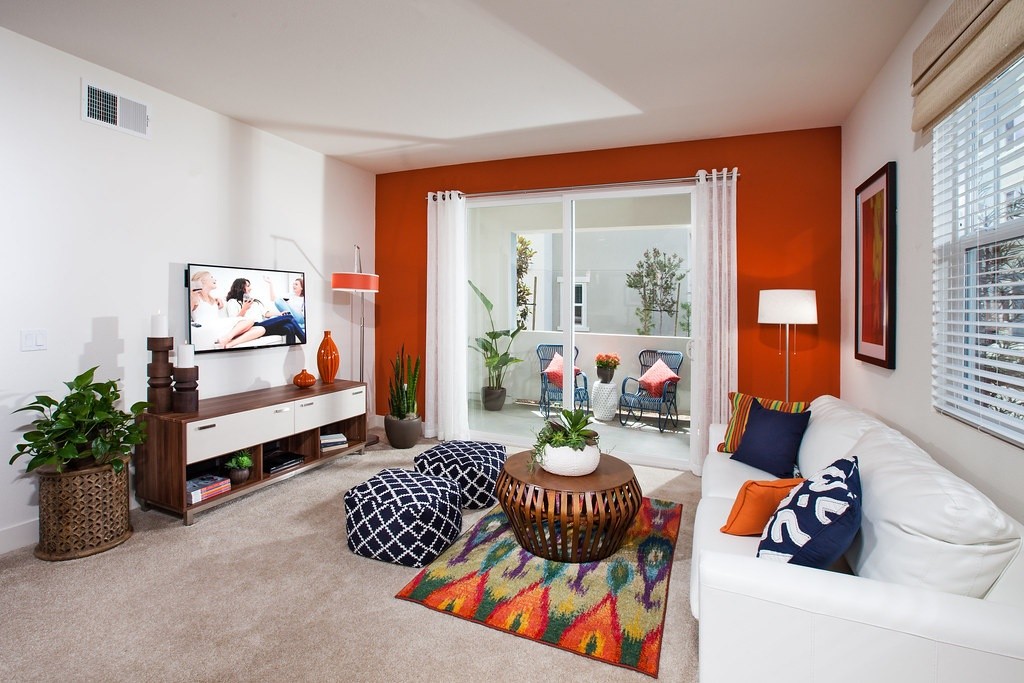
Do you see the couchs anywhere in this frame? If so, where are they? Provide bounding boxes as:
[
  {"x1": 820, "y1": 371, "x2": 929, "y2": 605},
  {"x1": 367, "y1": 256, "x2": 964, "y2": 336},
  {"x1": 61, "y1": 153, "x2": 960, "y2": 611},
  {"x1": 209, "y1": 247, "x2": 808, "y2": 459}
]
[{"x1": 689, "y1": 395, "x2": 1023, "y2": 683}]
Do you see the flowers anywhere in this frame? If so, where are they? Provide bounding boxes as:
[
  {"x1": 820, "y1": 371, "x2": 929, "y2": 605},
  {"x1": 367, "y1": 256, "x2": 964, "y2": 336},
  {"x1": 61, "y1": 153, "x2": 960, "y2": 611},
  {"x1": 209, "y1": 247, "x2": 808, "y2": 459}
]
[{"x1": 595, "y1": 353, "x2": 620, "y2": 367}]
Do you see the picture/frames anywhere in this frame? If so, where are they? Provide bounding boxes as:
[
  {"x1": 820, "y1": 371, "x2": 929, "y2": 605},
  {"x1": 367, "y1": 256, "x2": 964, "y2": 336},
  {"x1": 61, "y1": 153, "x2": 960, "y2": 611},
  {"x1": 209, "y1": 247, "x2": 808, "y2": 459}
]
[{"x1": 855, "y1": 161, "x2": 896, "y2": 370}]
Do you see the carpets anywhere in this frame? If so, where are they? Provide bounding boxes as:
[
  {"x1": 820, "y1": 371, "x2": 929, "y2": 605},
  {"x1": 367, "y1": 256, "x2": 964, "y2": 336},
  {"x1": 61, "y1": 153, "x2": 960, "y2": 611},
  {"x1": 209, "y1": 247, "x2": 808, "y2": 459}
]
[{"x1": 393, "y1": 495, "x2": 683, "y2": 679}]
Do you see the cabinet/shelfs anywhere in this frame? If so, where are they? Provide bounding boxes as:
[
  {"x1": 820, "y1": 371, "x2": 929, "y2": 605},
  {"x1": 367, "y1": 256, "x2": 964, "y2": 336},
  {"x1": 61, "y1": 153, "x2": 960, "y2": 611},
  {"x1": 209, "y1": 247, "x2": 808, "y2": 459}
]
[{"x1": 135, "y1": 378, "x2": 368, "y2": 526}]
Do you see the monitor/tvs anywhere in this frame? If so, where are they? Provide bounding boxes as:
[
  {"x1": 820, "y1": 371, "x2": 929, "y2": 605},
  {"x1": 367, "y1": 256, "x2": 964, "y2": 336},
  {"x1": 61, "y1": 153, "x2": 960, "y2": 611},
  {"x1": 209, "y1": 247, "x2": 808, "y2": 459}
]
[{"x1": 187, "y1": 262, "x2": 306, "y2": 354}]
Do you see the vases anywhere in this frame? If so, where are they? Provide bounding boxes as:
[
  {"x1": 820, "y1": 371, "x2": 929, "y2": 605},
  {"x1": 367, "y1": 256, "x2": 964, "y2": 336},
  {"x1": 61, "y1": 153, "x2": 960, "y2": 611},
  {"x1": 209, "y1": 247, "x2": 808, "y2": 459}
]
[{"x1": 597, "y1": 366, "x2": 614, "y2": 382}]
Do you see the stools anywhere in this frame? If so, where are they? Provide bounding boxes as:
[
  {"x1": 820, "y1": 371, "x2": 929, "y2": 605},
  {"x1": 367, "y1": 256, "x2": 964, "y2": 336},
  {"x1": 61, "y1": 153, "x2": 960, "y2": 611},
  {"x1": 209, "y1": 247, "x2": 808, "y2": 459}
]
[
  {"x1": 344, "y1": 467, "x2": 462, "y2": 568},
  {"x1": 413, "y1": 439, "x2": 507, "y2": 509}
]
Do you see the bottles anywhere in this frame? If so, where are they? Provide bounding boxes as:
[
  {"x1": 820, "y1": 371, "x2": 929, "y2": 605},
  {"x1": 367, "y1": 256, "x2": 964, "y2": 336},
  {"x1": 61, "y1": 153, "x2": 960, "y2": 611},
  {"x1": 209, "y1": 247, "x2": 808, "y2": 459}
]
[
  {"x1": 317, "y1": 331, "x2": 340, "y2": 385},
  {"x1": 293, "y1": 369, "x2": 316, "y2": 388}
]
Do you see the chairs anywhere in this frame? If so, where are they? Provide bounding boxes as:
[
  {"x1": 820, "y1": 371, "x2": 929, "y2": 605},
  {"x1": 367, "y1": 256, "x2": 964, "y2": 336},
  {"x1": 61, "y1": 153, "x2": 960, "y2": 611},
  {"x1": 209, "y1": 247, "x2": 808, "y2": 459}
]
[
  {"x1": 536, "y1": 343, "x2": 589, "y2": 420},
  {"x1": 619, "y1": 350, "x2": 684, "y2": 432}
]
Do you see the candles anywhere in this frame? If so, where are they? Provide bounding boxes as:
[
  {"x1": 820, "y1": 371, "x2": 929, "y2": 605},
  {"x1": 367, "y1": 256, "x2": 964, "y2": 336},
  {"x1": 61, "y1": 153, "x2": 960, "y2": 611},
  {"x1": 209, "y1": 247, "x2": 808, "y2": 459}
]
[
  {"x1": 151, "y1": 312, "x2": 168, "y2": 338},
  {"x1": 177, "y1": 339, "x2": 195, "y2": 368}
]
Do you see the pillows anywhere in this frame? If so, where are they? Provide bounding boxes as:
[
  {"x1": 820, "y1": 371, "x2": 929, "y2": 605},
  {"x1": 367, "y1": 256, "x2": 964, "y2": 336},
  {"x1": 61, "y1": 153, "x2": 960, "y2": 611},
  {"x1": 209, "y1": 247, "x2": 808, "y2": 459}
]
[
  {"x1": 542, "y1": 352, "x2": 580, "y2": 390},
  {"x1": 717, "y1": 391, "x2": 809, "y2": 453},
  {"x1": 720, "y1": 478, "x2": 805, "y2": 536},
  {"x1": 729, "y1": 397, "x2": 811, "y2": 479},
  {"x1": 637, "y1": 358, "x2": 681, "y2": 398},
  {"x1": 756, "y1": 456, "x2": 862, "y2": 570}
]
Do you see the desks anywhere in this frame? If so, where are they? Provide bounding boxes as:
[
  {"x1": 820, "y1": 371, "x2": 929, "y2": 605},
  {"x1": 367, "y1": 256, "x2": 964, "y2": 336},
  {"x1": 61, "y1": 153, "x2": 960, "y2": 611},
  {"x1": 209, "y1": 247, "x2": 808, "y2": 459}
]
[
  {"x1": 591, "y1": 380, "x2": 618, "y2": 421},
  {"x1": 31, "y1": 451, "x2": 134, "y2": 562},
  {"x1": 496, "y1": 450, "x2": 643, "y2": 563}
]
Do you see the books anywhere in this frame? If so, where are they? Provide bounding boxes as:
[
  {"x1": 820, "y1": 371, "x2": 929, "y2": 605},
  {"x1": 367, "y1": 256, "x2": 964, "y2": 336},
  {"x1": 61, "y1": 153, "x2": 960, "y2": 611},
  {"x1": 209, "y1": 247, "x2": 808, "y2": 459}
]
[
  {"x1": 186, "y1": 474, "x2": 232, "y2": 505},
  {"x1": 263, "y1": 447, "x2": 305, "y2": 474},
  {"x1": 320, "y1": 433, "x2": 348, "y2": 452}
]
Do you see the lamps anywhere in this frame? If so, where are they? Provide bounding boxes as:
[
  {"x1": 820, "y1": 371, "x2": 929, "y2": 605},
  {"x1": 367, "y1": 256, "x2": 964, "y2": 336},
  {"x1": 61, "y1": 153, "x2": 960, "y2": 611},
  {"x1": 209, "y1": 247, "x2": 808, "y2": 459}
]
[
  {"x1": 331, "y1": 243, "x2": 380, "y2": 447},
  {"x1": 757, "y1": 288, "x2": 818, "y2": 402}
]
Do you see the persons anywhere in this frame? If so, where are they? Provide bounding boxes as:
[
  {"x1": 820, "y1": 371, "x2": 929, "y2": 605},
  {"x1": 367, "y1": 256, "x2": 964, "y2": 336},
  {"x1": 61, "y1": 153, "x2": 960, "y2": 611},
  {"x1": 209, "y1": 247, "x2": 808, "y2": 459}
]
[
  {"x1": 226, "y1": 278, "x2": 306, "y2": 345},
  {"x1": 263, "y1": 275, "x2": 305, "y2": 329},
  {"x1": 190, "y1": 271, "x2": 266, "y2": 349}
]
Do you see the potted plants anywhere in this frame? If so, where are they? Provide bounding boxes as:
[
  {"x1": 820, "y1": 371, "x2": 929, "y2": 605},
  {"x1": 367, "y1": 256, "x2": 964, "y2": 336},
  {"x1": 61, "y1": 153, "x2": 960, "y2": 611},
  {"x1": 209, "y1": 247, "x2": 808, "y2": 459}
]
[
  {"x1": 467, "y1": 280, "x2": 525, "y2": 412},
  {"x1": 224, "y1": 448, "x2": 254, "y2": 483},
  {"x1": 383, "y1": 343, "x2": 422, "y2": 449},
  {"x1": 8, "y1": 364, "x2": 160, "y2": 474},
  {"x1": 525, "y1": 408, "x2": 601, "y2": 478}
]
